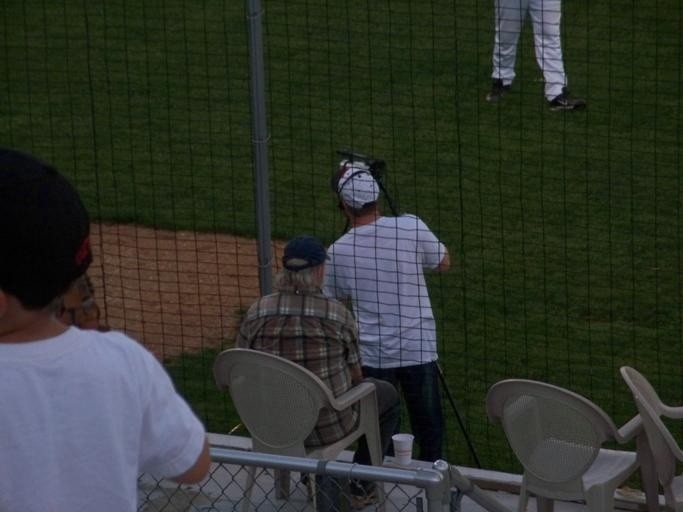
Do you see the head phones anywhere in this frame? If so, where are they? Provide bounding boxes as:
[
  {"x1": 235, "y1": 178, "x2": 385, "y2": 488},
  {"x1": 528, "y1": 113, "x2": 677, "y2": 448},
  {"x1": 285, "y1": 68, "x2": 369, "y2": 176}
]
[{"x1": 336, "y1": 171, "x2": 368, "y2": 210}]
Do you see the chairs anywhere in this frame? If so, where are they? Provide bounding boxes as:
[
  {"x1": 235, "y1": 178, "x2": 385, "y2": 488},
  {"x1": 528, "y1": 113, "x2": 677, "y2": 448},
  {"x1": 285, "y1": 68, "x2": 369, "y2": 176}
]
[
  {"x1": 484, "y1": 366, "x2": 683, "y2": 511},
  {"x1": 212, "y1": 344, "x2": 387, "y2": 512}
]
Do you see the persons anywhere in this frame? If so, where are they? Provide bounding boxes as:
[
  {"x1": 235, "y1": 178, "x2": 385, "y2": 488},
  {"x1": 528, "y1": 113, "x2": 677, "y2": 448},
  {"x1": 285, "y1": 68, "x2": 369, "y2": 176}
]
[
  {"x1": 235, "y1": 234, "x2": 402, "y2": 510},
  {"x1": 0, "y1": 148, "x2": 213, "y2": 512},
  {"x1": 484, "y1": 1, "x2": 588, "y2": 116},
  {"x1": 319, "y1": 155, "x2": 453, "y2": 465}
]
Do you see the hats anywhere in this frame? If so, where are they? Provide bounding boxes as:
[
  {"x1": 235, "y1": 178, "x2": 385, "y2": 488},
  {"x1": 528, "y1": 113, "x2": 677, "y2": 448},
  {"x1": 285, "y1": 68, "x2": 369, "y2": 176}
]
[
  {"x1": 339, "y1": 167, "x2": 380, "y2": 209},
  {"x1": 281, "y1": 234, "x2": 332, "y2": 270},
  {"x1": 0, "y1": 151, "x2": 93, "y2": 291}
]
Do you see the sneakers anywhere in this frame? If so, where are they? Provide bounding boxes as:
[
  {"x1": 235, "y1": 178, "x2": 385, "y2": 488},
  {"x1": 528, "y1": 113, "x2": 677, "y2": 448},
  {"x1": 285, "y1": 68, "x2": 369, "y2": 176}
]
[
  {"x1": 347, "y1": 480, "x2": 379, "y2": 512},
  {"x1": 487, "y1": 78, "x2": 511, "y2": 101},
  {"x1": 548, "y1": 88, "x2": 586, "y2": 111}
]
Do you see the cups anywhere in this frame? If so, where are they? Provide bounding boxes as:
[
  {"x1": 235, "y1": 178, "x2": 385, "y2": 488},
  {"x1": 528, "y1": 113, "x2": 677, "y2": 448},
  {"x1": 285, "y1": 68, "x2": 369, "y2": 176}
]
[{"x1": 391, "y1": 433, "x2": 415, "y2": 466}]
